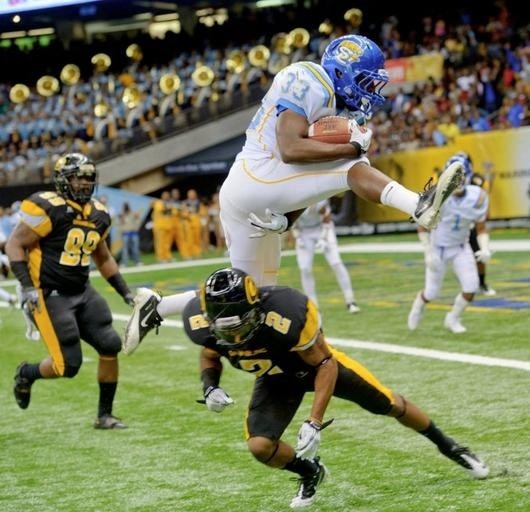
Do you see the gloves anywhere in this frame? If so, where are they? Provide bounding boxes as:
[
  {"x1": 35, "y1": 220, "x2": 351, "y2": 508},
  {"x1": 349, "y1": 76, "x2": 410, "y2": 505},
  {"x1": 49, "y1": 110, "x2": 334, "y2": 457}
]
[
  {"x1": 417, "y1": 232, "x2": 442, "y2": 271},
  {"x1": 350, "y1": 120, "x2": 373, "y2": 154},
  {"x1": 11, "y1": 260, "x2": 40, "y2": 311},
  {"x1": 248, "y1": 208, "x2": 288, "y2": 239},
  {"x1": 196, "y1": 386, "x2": 234, "y2": 412},
  {"x1": 316, "y1": 222, "x2": 334, "y2": 254},
  {"x1": 107, "y1": 274, "x2": 135, "y2": 306},
  {"x1": 474, "y1": 233, "x2": 492, "y2": 263},
  {"x1": 291, "y1": 226, "x2": 306, "y2": 250},
  {"x1": 296, "y1": 417, "x2": 334, "y2": 462}
]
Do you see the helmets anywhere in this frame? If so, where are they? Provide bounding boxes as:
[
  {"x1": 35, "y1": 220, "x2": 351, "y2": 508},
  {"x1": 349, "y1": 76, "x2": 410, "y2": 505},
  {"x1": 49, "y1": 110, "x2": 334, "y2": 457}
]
[
  {"x1": 321, "y1": 35, "x2": 389, "y2": 117},
  {"x1": 201, "y1": 267, "x2": 262, "y2": 346},
  {"x1": 445, "y1": 156, "x2": 472, "y2": 182},
  {"x1": 53, "y1": 152, "x2": 96, "y2": 204}
]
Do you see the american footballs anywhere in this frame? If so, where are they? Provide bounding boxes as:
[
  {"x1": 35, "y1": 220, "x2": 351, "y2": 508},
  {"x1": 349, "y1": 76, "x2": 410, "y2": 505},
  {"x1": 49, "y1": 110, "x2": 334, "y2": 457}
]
[{"x1": 307, "y1": 116, "x2": 364, "y2": 144}]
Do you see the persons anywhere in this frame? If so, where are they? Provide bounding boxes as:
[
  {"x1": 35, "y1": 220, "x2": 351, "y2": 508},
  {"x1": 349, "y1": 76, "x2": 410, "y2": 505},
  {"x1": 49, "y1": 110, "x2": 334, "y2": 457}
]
[
  {"x1": 0, "y1": 0, "x2": 530, "y2": 307},
  {"x1": 408, "y1": 150, "x2": 492, "y2": 334},
  {"x1": 180, "y1": 267, "x2": 489, "y2": 508},
  {"x1": 5, "y1": 151, "x2": 134, "y2": 429},
  {"x1": 291, "y1": 198, "x2": 361, "y2": 319},
  {"x1": 120, "y1": 31, "x2": 471, "y2": 359}
]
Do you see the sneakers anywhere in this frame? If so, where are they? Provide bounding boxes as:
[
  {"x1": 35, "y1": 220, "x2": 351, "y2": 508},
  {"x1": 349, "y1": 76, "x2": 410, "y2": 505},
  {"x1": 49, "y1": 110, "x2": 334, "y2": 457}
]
[
  {"x1": 408, "y1": 291, "x2": 425, "y2": 330},
  {"x1": 448, "y1": 444, "x2": 491, "y2": 480},
  {"x1": 443, "y1": 314, "x2": 465, "y2": 333},
  {"x1": 94, "y1": 415, "x2": 128, "y2": 429},
  {"x1": 347, "y1": 304, "x2": 360, "y2": 312},
  {"x1": 289, "y1": 456, "x2": 329, "y2": 507},
  {"x1": 412, "y1": 162, "x2": 466, "y2": 229},
  {"x1": 124, "y1": 287, "x2": 163, "y2": 356},
  {"x1": 14, "y1": 361, "x2": 33, "y2": 409},
  {"x1": 482, "y1": 289, "x2": 495, "y2": 297}
]
